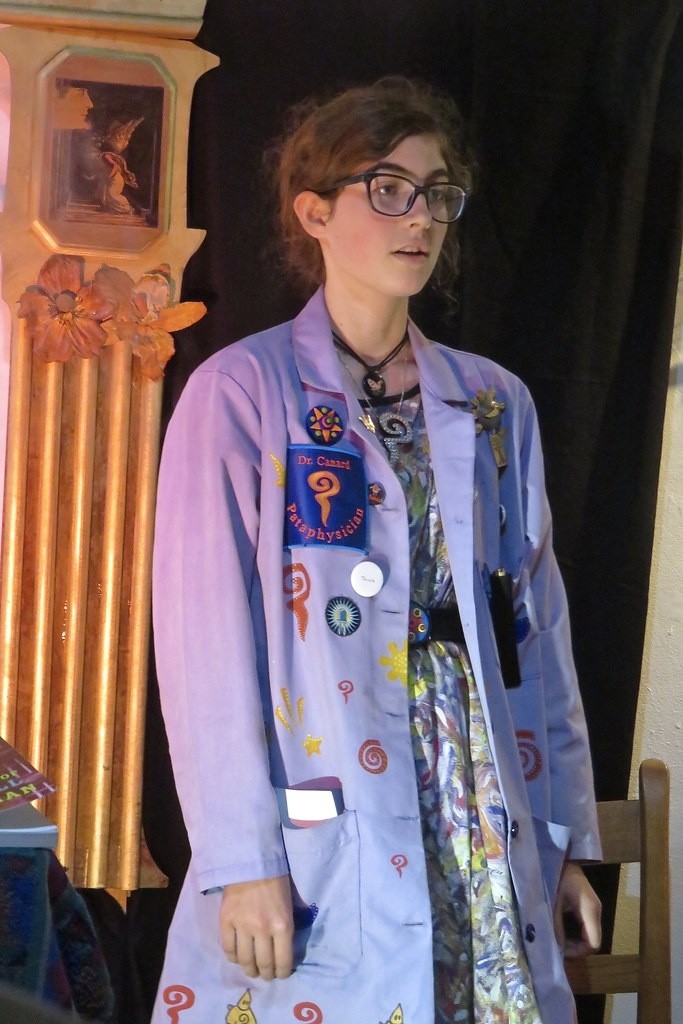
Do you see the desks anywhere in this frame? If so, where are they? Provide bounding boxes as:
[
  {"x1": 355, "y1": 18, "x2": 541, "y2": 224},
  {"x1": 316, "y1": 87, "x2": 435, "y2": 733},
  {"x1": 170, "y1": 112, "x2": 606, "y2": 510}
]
[{"x1": 0, "y1": 803, "x2": 59, "y2": 1024}]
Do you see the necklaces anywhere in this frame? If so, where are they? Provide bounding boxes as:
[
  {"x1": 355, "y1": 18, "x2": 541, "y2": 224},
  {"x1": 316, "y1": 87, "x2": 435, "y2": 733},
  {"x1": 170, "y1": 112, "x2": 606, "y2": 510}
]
[{"x1": 331, "y1": 330, "x2": 413, "y2": 470}]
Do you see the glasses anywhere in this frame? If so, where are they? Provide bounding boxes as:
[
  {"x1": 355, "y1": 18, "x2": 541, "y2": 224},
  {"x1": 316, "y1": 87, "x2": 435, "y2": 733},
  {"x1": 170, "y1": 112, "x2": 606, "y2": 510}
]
[{"x1": 325, "y1": 171, "x2": 466, "y2": 224}]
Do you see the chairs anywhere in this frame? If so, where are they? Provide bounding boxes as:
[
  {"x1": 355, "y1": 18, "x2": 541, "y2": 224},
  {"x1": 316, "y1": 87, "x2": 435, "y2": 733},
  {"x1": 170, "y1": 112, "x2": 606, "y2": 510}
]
[{"x1": 560, "y1": 757, "x2": 674, "y2": 1024}]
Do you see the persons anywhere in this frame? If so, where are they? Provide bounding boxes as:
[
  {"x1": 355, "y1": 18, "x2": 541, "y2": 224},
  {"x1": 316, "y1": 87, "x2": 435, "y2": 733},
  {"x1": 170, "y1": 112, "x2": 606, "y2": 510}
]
[{"x1": 149, "y1": 74, "x2": 603, "y2": 1024}]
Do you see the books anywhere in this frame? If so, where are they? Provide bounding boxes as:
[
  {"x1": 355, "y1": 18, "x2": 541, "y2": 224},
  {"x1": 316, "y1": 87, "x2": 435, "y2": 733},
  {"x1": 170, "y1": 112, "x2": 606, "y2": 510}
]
[{"x1": 0, "y1": 730, "x2": 60, "y2": 849}]
